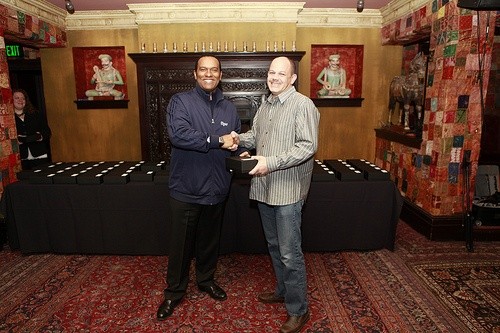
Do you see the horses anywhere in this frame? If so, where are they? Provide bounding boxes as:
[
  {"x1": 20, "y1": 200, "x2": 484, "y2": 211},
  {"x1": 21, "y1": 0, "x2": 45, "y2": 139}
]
[{"x1": 388, "y1": 51, "x2": 426, "y2": 130}]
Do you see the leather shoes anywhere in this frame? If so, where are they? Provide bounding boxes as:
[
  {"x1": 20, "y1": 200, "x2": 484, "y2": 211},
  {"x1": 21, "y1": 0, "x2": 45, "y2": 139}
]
[
  {"x1": 278, "y1": 311, "x2": 309, "y2": 333},
  {"x1": 156, "y1": 298, "x2": 182, "y2": 320},
  {"x1": 258, "y1": 293, "x2": 285, "y2": 303},
  {"x1": 197, "y1": 283, "x2": 227, "y2": 301}
]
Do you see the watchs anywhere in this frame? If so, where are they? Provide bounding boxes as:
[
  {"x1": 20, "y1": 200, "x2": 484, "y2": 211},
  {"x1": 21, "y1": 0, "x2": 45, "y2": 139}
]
[{"x1": 218, "y1": 136, "x2": 224, "y2": 147}]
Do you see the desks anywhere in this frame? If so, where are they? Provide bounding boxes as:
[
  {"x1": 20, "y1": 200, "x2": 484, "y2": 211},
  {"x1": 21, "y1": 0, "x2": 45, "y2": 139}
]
[{"x1": 4, "y1": 159, "x2": 404, "y2": 255}]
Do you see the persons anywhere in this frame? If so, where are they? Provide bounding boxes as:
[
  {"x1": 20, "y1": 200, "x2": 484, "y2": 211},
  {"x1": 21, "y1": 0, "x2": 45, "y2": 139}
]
[
  {"x1": 157, "y1": 55, "x2": 253, "y2": 320},
  {"x1": 13, "y1": 90, "x2": 52, "y2": 170},
  {"x1": 85, "y1": 54, "x2": 125, "y2": 100},
  {"x1": 316, "y1": 54, "x2": 352, "y2": 98},
  {"x1": 227, "y1": 56, "x2": 321, "y2": 333}
]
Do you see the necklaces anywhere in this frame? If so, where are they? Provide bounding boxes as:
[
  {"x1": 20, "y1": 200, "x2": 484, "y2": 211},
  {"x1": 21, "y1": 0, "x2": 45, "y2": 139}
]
[{"x1": 16, "y1": 112, "x2": 24, "y2": 117}]
